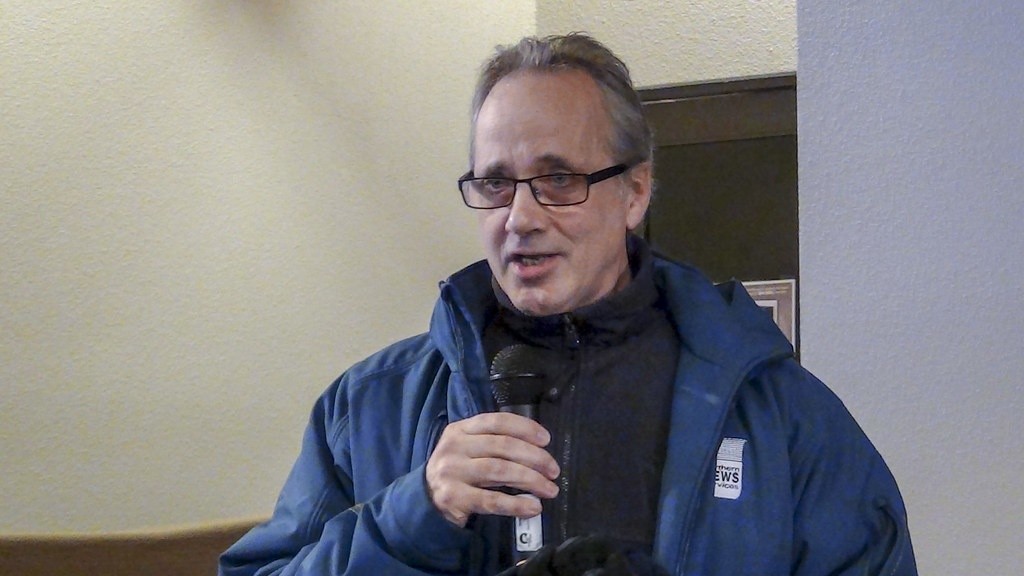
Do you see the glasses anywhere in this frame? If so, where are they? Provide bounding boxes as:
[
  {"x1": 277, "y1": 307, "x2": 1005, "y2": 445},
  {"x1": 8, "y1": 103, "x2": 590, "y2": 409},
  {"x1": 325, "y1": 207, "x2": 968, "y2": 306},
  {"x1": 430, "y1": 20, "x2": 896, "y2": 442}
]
[{"x1": 458, "y1": 159, "x2": 639, "y2": 209}]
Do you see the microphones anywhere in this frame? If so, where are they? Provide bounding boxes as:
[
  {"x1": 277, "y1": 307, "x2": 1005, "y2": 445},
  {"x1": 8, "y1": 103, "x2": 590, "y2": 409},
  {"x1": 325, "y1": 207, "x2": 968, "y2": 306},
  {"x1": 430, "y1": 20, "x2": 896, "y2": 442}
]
[{"x1": 492, "y1": 345, "x2": 548, "y2": 560}]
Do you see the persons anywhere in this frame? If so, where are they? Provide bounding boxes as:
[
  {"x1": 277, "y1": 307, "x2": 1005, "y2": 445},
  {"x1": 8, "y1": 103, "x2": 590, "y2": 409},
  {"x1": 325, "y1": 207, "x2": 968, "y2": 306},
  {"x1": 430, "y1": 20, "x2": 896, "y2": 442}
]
[{"x1": 217, "y1": 33, "x2": 918, "y2": 576}]
